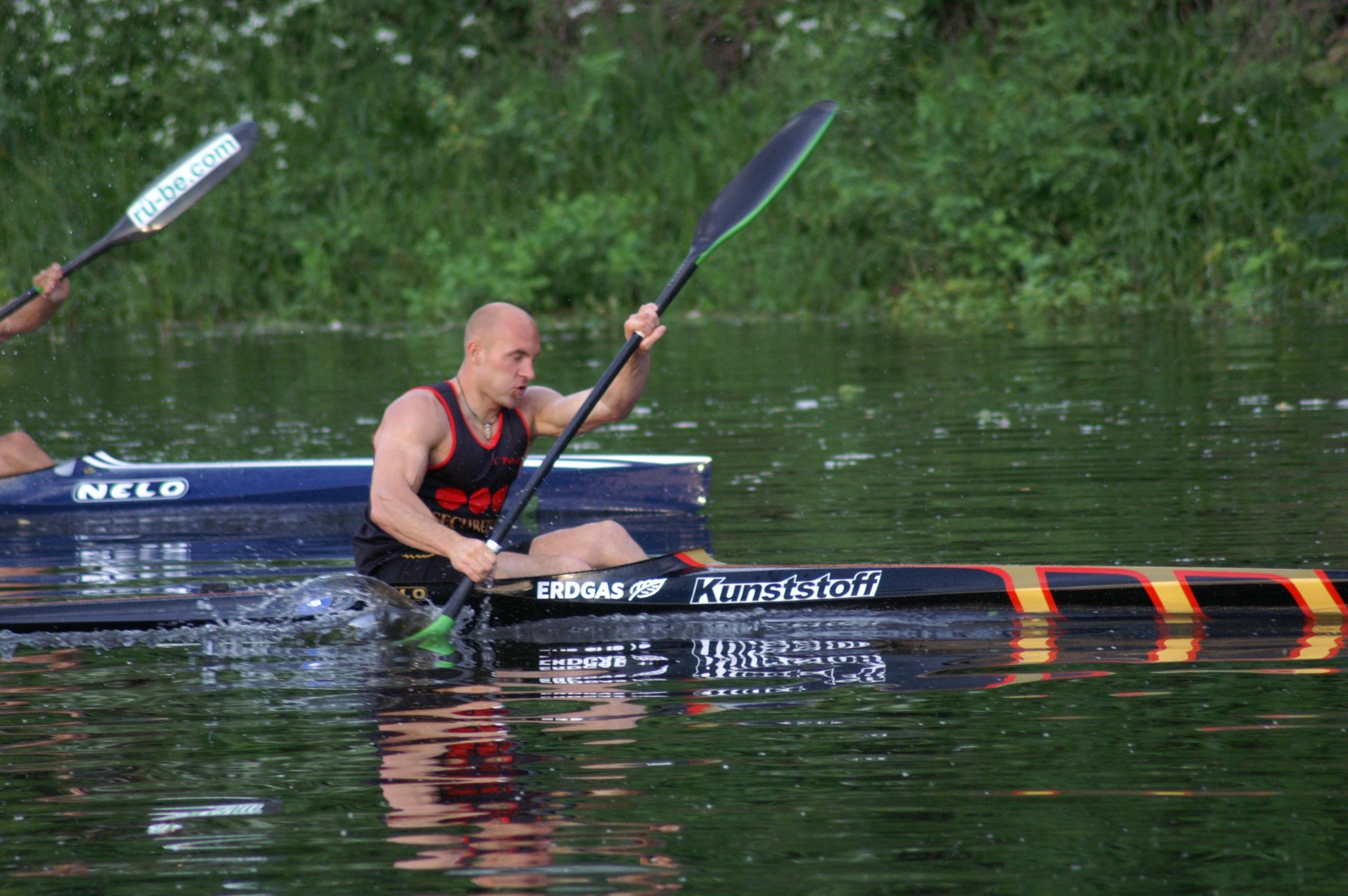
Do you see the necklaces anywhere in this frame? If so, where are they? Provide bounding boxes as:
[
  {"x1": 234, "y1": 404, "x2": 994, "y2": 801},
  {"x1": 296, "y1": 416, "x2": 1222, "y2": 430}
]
[{"x1": 457, "y1": 375, "x2": 502, "y2": 440}]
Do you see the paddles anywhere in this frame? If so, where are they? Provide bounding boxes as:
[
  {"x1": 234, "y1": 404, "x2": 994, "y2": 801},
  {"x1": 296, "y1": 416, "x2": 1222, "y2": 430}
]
[
  {"x1": 0, "y1": 116, "x2": 264, "y2": 319},
  {"x1": 391, "y1": 94, "x2": 843, "y2": 640}
]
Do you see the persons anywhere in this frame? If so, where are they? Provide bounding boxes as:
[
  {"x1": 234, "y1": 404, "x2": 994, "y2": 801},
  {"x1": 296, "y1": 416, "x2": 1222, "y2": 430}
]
[
  {"x1": 0, "y1": 262, "x2": 71, "y2": 477},
  {"x1": 351, "y1": 299, "x2": 667, "y2": 616}
]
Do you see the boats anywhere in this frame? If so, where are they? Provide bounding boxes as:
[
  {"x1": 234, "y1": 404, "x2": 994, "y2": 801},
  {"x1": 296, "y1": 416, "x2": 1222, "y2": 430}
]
[
  {"x1": 0, "y1": 544, "x2": 1348, "y2": 619},
  {"x1": 0, "y1": 446, "x2": 713, "y2": 510}
]
